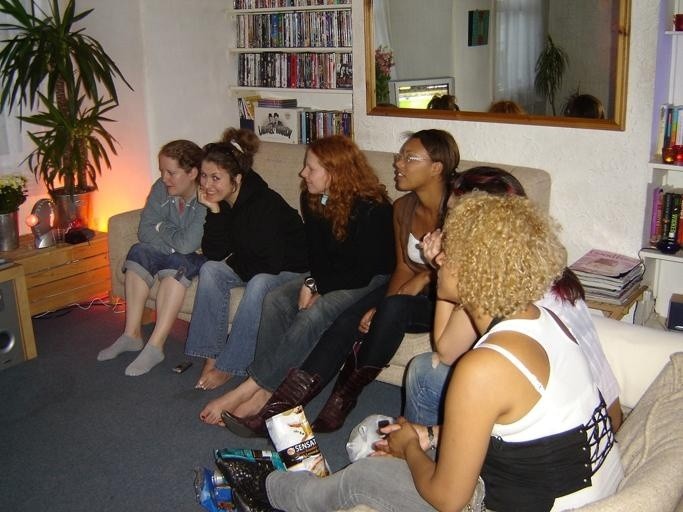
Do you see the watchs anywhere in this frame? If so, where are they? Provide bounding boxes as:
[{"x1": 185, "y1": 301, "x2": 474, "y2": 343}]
[{"x1": 305, "y1": 275, "x2": 318, "y2": 294}]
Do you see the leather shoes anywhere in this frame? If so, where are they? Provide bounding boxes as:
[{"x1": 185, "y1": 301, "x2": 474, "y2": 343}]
[{"x1": 215, "y1": 455, "x2": 286, "y2": 512}]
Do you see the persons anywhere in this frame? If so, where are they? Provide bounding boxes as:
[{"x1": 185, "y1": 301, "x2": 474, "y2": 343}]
[
  {"x1": 95, "y1": 138, "x2": 207, "y2": 378},
  {"x1": 180, "y1": 126, "x2": 317, "y2": 391},
  {"x1": 211, "y1": 191, "x2": 626, "y2": 511},
  {"x1": 427, "y1": 93, "x2": 461, "y2": 110},
  {"x1": 220, "y1": 128, "x2": 460, "y2": 441},
  {"x1": 378, "y1": 101, "x2": 398, "y2": 108},
  {"x1": 196, "y1": 132, "x2": 395, "y2": 429},
  {"x1": 485, "y1": 99, "x2": 529, "y2": 116},
  {"x1": 401, "y1": 166, "x2": 626, "y2": 437},
  {"x1": 561, "y1": 88, "x2": 607, "y2": 118}
]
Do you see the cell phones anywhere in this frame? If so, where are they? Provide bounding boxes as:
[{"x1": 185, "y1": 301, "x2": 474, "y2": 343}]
[{"x1": 172, "y1": 360, "x2": 193, "y2": 373}]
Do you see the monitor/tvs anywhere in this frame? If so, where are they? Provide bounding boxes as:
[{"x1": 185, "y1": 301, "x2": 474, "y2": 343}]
[{"x1": 389, "y1": 76, "x2": 455, "y2": 110}]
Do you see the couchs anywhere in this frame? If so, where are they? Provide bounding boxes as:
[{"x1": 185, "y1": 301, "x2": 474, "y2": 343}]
[{"x1": 105, "y1": 132, "x2": 551, "y2": 389}]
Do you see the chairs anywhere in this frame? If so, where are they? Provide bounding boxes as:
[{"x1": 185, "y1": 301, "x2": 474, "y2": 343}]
[{"x1": 575, "y1": 348, "x2": 681, "y2": 512}]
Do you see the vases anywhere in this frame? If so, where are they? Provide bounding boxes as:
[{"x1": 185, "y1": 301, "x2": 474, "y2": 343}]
[
  {"x1": 0, "y1": 212, "x2": 21, "y2": 252},
  {"x1": 375, "y1": 78, "x2": 389, "y2": 106}
]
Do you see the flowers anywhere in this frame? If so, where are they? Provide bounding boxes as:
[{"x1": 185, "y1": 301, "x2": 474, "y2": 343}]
[
  {"x1": 0, "y1": 174, "x2": 30, "y2": 214},
  {"x1": 373, "y1": 44, "x2": 394, "y2": 100}
]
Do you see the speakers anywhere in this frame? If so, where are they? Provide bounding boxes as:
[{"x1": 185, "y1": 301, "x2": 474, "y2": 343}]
[{"x1": 0, "y1": 257, "x2": 39, "y2": 373}]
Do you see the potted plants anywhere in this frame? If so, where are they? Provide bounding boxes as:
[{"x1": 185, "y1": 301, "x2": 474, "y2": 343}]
[{"x1": 0, "y1": 0, "x2": 134, "y2": 231}]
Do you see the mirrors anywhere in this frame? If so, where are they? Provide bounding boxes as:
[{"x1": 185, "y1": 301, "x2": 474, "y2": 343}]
[{"x1": 360, "y1": 1, "x2": 632, "y2": 134}]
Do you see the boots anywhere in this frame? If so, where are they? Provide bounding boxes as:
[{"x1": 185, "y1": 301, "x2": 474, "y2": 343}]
[
  {"x1": 221, "y1": 367, "x2": 327, "y2": 438},
  {"x1": 308, "y1": 343, "x2": 389, "y2": 434}
]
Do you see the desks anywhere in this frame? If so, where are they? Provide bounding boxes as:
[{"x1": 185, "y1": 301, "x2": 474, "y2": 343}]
[{"x1": 583, "y1": 283, "x2": 650, "y2": 323}]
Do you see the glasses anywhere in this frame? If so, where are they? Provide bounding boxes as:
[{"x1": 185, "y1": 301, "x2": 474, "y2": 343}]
[{"x1": 392, "y1": 153, "x2": 431, "y2": 164}]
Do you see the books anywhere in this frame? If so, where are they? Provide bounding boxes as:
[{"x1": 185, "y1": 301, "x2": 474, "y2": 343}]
[
  {"x1": 565, "y1": 100, "x2": 683, "y2": 330},
  {"x1": 232, "y1": 0, "x2": 354, "y2": 145}
]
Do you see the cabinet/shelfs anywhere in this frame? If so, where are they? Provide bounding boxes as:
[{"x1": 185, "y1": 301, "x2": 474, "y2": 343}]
[
  {"x1": 0, "y1": 228, "x2": 111, "y2": 319},
  {"x1": 230, "y1": 0, "x2": 354, "y2": 144},
  {"x1": 639, "y1": 156, "x2": 682, "y2": 330}
]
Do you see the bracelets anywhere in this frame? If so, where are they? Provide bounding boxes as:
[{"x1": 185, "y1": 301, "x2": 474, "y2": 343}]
[{"x1": 425, "y1": 423, "x2": 437, "y2": 450}]
